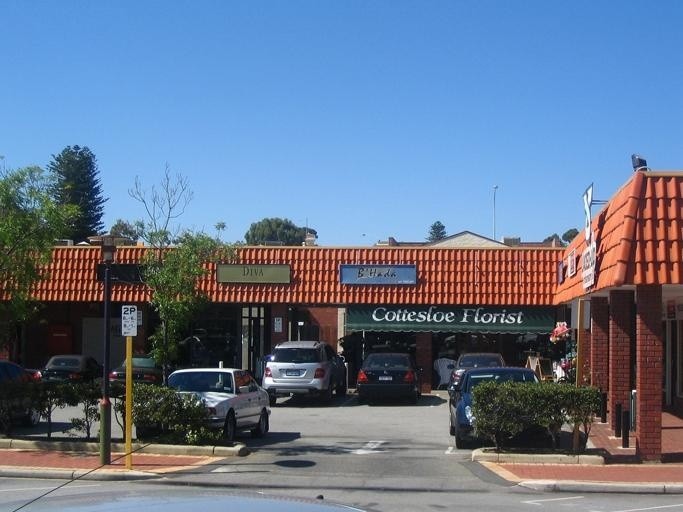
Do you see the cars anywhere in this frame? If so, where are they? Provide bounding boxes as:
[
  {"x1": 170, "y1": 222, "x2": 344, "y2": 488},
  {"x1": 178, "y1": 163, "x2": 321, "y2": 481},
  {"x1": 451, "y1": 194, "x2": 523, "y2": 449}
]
[
  {"x1": 260, "y1": 338, "x2": 426, "y2": 408},
  {"x1": 448, "y1": 350, "x2": 548, "y2": 452},
  {"x1": 147, "y1": 366, "x2": 270, "y2": 445},
  {"x1": 44, "y1": 354, "x2": 164, "y2": 390},
  {"x1": 0, "y1": 362, "x2": 45, "y2": 426}
]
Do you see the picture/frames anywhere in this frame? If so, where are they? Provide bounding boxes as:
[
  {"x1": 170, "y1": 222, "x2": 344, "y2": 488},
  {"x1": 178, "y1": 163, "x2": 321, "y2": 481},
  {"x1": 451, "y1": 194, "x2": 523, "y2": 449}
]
[{"x1": 567, "y1": 248, "x2": 577, "y2": 278}]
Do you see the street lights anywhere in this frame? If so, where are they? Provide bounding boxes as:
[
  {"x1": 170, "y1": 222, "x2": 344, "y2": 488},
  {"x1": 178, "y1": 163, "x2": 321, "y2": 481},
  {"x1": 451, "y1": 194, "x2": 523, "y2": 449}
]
[
  {"x1": 492, "y1": 185, "x2": 499, "y2": 240},
  {"x1": 100, "y1": 234, "x2": 116, "y2": 464}
]
